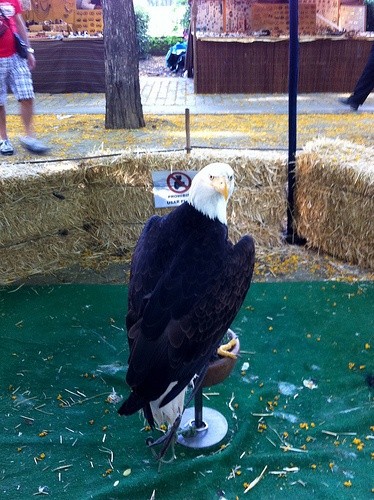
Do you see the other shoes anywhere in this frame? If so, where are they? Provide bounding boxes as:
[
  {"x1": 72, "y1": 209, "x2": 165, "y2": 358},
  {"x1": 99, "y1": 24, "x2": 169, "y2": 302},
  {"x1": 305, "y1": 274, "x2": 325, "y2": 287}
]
[{"x1": 338, "y1": 97, "x2": 359, "y2": 111}]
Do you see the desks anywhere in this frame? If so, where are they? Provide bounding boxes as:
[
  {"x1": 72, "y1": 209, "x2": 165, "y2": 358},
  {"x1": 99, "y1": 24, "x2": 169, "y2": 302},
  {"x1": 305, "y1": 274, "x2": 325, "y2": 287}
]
[
  {"x1": 195, "y1": 39, "x2": 374, "y2": 92},
  {"x1": 30, "y1": 40, "x2": 104, "y2": 94}
]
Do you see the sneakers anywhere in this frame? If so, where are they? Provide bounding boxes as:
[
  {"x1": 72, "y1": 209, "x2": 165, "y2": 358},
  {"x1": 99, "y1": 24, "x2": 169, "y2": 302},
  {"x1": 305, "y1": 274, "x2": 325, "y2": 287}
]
[
  {"x1": 1, "y1": 140, "x2": 14, "y2": 155},
  {"x1": 19, "y1": 136, "x2": 51, "y2": 153}
]
